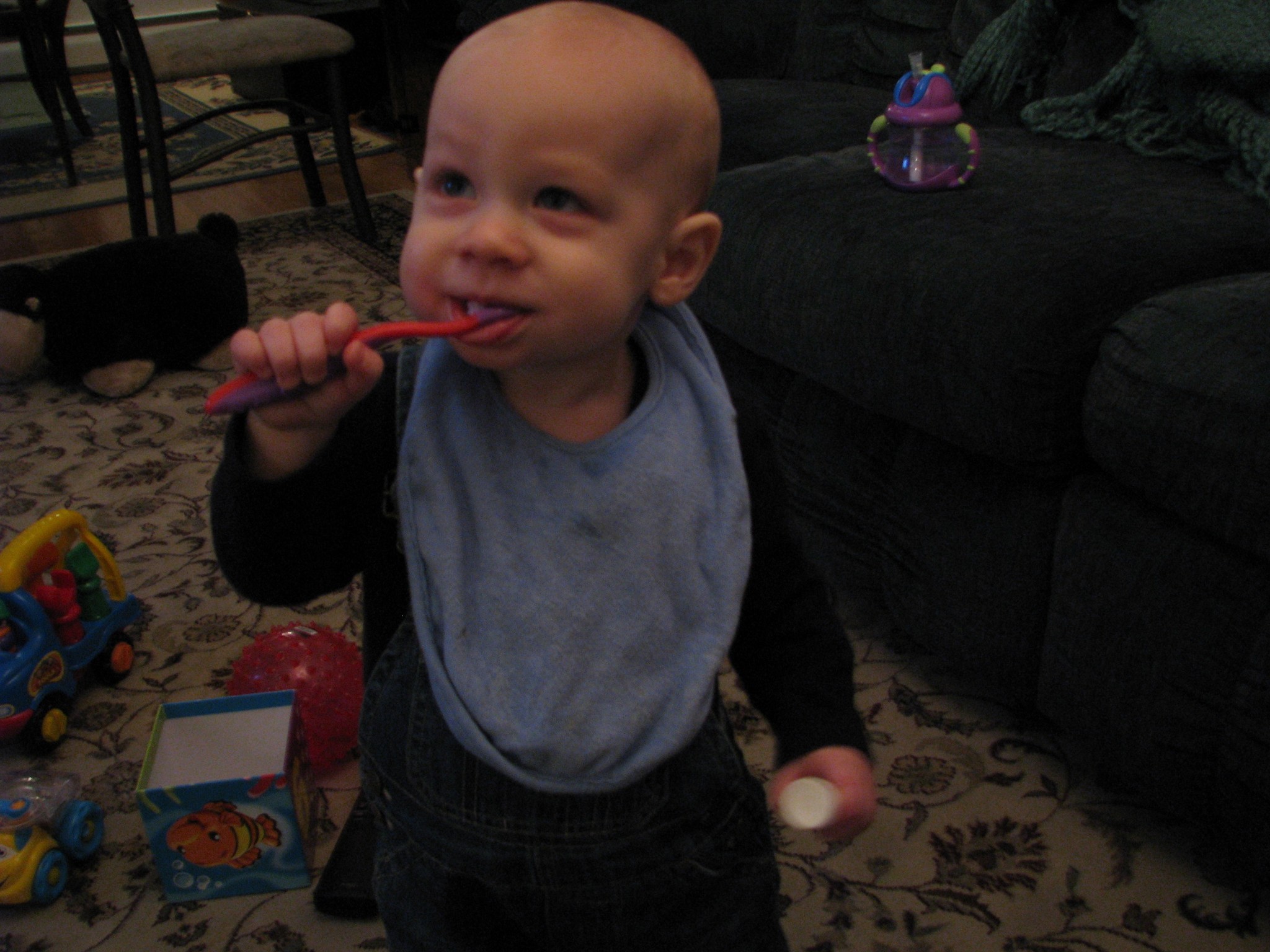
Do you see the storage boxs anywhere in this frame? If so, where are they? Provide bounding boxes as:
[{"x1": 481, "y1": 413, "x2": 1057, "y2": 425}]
[{"x1": 134, "y1": 688, "x2": 327, "y2": 904}]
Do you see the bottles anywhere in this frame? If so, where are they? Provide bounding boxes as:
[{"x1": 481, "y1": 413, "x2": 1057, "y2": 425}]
[{"x1": 866, "y1": 51, "x2": 982, "y2": 192}]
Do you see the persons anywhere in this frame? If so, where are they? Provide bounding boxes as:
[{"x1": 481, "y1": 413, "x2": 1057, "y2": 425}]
[{"x1": 210, "y1": 2, "x2": 876, "y2": 952}]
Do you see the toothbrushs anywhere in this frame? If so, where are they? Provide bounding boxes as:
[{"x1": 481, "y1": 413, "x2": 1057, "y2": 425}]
[{"x1": 201, "y1": 305, "x2": 518, "y2": 416}]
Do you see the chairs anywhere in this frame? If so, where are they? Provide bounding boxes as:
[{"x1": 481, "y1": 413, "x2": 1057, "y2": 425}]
[
  {"x1": 0, "y1": 0, "x2": 98, "y2": 190},
  {"x1": 85, "y1": 0, "x2": 382, "y2": 252}
]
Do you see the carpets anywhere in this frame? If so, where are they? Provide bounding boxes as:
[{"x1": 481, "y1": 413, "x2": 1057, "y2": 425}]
[
  {"x1": 0, "y1": 74, "x2": 398, "y2": 225},
  {"x1": 0, "y1": 180, "x2": 1270, "y2": 952}
]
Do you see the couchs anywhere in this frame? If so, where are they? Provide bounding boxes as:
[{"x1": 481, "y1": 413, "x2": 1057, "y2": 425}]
[{"x1": 254, "y1": 1, "x2": 1268, "y2": 907}]
